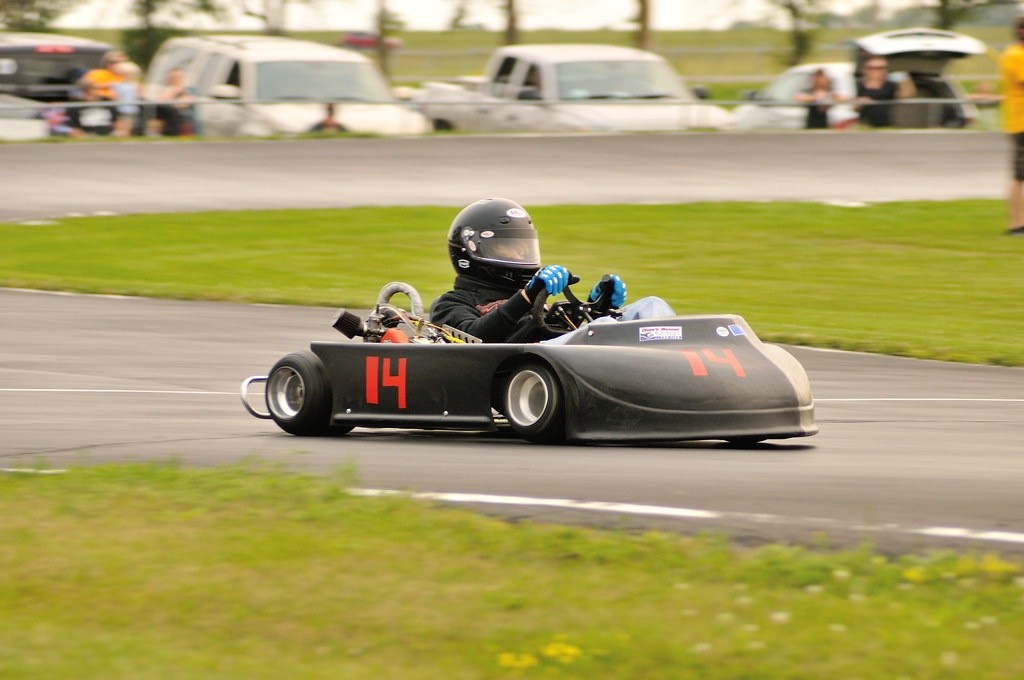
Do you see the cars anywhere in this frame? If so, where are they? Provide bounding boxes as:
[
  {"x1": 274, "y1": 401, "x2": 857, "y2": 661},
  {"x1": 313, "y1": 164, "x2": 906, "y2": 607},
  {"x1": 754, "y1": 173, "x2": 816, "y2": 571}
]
[{"x1": 731, "y1": 24, "x2": 988, "y2": 126}]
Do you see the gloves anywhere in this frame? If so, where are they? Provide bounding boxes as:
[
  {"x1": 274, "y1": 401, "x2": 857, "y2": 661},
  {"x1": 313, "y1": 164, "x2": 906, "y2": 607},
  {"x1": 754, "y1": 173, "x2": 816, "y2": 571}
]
[
  {"x1": 524, "y1": 264, "x2": 580, "y2": 301},
  {"x1": 586, "y1": 274, "x2": 627, "y2": 318}
]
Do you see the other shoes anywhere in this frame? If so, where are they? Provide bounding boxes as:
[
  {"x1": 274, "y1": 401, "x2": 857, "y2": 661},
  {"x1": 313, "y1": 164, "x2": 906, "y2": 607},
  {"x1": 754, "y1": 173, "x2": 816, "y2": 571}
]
[{"x1": 1004, "y1": 225, "x2": 1024, "y2": 234}]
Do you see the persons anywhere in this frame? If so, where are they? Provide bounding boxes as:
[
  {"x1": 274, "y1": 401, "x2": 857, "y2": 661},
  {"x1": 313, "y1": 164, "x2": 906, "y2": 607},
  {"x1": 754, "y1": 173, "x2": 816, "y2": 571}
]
[
  {"x1": 796, "y1": 53, "x2": 917, "y2": 131},
  {"x1": 428, "y1": 197, "x2": 627, "y2": 344},
  {"x1": 35, "y1": 48, "x2": 348, "y2": 141},
  {"x1": 998, "y1": 13, "x2": 1024, "y2": 233}
]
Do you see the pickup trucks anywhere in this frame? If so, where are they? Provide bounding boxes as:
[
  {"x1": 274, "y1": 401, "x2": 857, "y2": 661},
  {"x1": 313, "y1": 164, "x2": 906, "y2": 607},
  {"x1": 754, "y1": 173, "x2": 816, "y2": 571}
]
[
  {"x1": 413, "y1": 41, "x2": 730, "y2": 135},
  {"x1": 0, "y1": 33, "x2": 202, "y2": 145}
]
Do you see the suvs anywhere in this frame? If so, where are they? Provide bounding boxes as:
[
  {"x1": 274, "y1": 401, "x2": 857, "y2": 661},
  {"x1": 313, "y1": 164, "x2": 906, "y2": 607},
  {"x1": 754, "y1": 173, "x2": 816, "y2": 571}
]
[{"x1": 142, "y1": 32, "x2": 429, "y2": 141}]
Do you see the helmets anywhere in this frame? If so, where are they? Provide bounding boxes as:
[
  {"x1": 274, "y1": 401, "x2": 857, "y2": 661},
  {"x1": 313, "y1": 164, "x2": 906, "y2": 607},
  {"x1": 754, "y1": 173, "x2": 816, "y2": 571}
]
[{"x1": 447, "y1": 197, "x2": 541, "y2": 291}]
